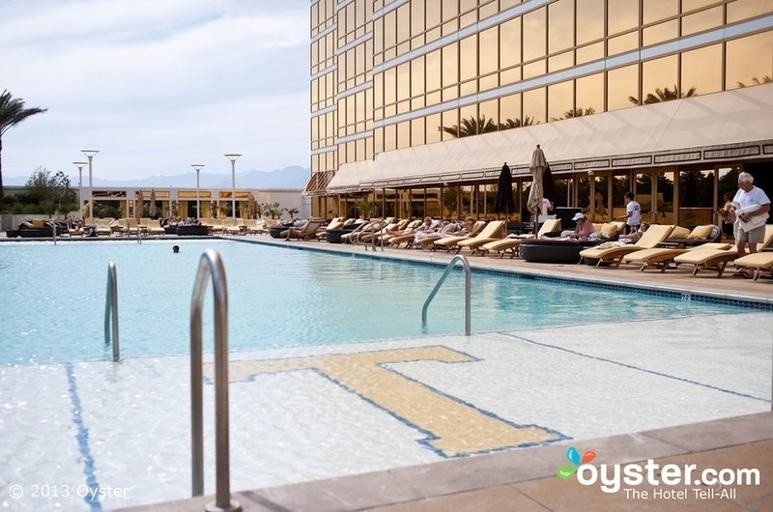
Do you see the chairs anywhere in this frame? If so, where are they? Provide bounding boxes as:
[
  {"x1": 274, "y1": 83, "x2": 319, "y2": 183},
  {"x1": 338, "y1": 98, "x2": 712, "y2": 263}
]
[
  {"x1": 269, "y1": 211, "x2": 772, "y2": 300},
  {"x1": 5, "y1": 215, "x2": 269, "y2": 242}
]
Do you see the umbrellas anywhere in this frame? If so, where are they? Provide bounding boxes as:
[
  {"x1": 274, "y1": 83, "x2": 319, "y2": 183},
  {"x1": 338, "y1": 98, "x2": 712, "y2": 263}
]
[
  {"x1": 527, "y1": 144, "x2": 548, "y2": 238},
  {"x1": 595, "y1": 191, "x2": 604, "y2": 220},
  {"x1": 246, "y1": 193, "x2": 255, "y2": 220},
  {"x1": 149, "y1": 189, "x2": 156, "y2": 219},
  {"x1": 137, "y1": 188, "x2": 144, "y2": 218}
]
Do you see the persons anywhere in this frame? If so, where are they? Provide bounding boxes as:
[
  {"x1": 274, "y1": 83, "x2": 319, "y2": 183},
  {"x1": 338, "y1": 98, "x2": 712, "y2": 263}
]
[
  {"x1": 569, "y1": 213, "x2": 594, "y2": 240},
  {"x1": 533, "y1": 197, "x2": 553, "y2": 233},
  {"x1": 732, "y1": 172, "x2": 770, "y2": 278},
  {"x1": 172, "y1": 246, "x2": 179, "y2": 252},
  {"x1": 618, "y1": 192, "x2": 641, "y2": 235},
  {"x1": 616, "y1": 224, "x2": 649, "y2": 243},
  {"x1": 722, "y1": 191, "x2": 734, "y2": 243},
  {"x1": 47, "y1": 215, "x2": 200, "y2": 231},
  {"x1": 261, "y1": 216, "x2": 474, "y2": 237}
]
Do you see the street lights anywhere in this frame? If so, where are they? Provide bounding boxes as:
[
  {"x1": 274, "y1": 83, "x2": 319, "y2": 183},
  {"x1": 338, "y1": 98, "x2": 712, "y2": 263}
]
[
  {"x1": 224, "y1": 154, "x2": 242, "y2": 223},
  {"x1": 72, "y1": 161, "x2": 88, "y2": 221},
  {"x1": 191, "y1": 164, "x2": 206, "y2": 221},
  {"x1": 80, "y1": 149, "x2": 98, "y2": 227}
]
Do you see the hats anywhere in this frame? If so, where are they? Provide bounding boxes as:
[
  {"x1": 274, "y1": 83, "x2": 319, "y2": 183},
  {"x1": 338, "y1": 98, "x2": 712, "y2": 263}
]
[{"x1": 571, "y1": 213, "x2": 585, "y2": 220}]
